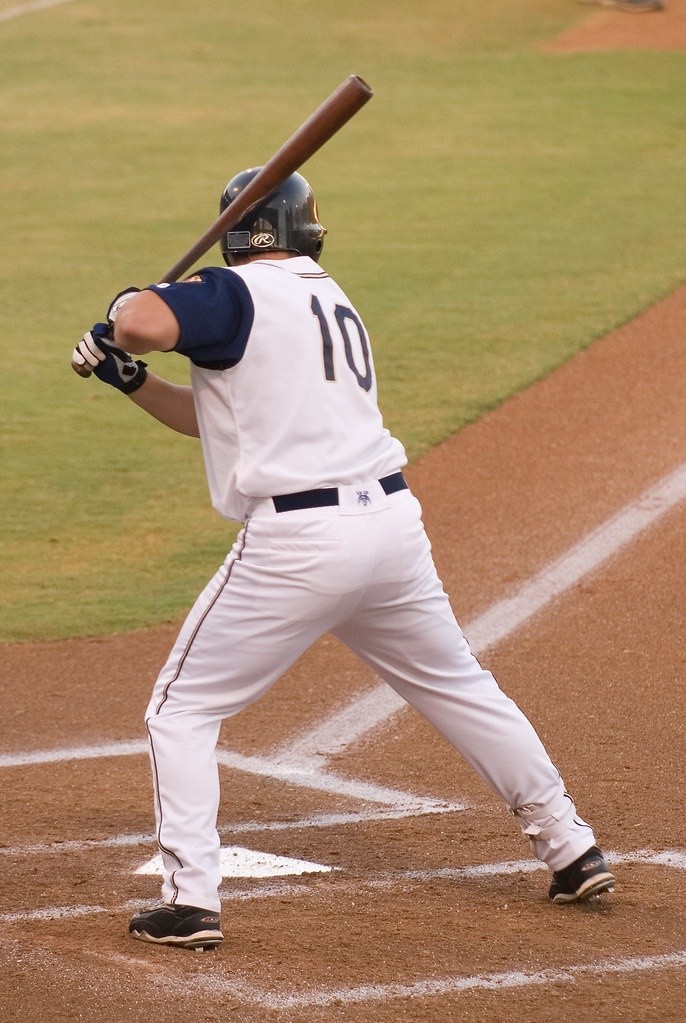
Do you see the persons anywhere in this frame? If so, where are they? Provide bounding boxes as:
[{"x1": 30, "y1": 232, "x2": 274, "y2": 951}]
[{"x1": 72, "y1": 169, "x2": 614, "y2": 946}]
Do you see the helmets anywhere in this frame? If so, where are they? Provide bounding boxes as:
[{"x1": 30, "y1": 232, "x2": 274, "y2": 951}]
[{"x1": 218, "y1": 166, "x2": 327, "y2": 264}]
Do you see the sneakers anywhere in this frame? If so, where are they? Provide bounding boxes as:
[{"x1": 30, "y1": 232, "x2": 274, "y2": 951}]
[
  {"x1": 129, "y1": 905, "x2": 225, "y2": 953},
  {"x1": 549, "y1": 845, "x2": 616, "y2": 904}
]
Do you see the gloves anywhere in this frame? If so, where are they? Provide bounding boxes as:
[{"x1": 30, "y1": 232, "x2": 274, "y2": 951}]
[
  {"x1": 71, "y1": 323, "x2": 148, "y2": 395},
  {"x1": 107, "y1": 287, "x2": 142, "y2": 332}
]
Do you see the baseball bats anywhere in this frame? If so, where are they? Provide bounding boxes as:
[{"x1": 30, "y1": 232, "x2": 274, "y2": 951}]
[{"x1": 71, "y1": 73, "x2": 375, "y2": 376}]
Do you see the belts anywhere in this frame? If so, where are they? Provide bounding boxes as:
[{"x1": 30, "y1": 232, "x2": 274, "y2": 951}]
[{"x1": 272, "y1": 471, "x2": 409, "y2": 513}]
